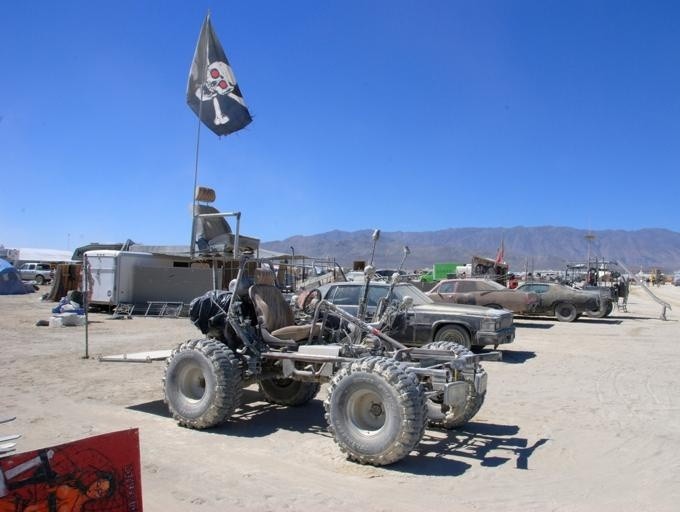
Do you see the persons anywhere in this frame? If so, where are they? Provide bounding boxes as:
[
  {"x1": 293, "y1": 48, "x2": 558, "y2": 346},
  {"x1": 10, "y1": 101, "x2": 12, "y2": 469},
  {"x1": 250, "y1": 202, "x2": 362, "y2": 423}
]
[{"x1": 1, "y1": 470, "x2": 117, "y2": 512}]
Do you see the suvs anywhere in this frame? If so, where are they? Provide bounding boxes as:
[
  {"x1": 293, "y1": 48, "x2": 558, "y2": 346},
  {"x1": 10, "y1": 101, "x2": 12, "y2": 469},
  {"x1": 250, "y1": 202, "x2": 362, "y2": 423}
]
[
  {"x1": 301, "y1": 277, "x2": 518, "y2": 351},
  {"x1": 161, "y1": 227, "x2": 503, "y2": 466}
]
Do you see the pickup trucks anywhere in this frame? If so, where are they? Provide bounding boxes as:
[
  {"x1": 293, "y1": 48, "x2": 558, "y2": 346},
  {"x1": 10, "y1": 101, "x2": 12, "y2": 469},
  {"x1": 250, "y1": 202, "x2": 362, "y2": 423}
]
[{"x1": 16, "y1": 262, "x2": 56, "y2": 285}]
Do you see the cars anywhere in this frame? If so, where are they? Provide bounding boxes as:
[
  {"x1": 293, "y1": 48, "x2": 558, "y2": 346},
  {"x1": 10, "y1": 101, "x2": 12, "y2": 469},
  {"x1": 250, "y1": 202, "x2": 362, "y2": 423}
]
[
  {"x1": 421, "y1": 278, "x2": 542, "y2": 322},
  {"x1": 510, "y1": 258, "x2": 680, "y2": 322},
  {"x1": 259, "y1": 261, "x2": 412, "y2": 292}
]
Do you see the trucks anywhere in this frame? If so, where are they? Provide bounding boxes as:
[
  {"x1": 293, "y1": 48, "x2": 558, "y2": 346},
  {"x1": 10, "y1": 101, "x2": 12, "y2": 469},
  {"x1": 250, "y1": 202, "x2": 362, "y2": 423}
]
[{"x1": 420, "y1": 262, "x2": 464, "y2": 280}]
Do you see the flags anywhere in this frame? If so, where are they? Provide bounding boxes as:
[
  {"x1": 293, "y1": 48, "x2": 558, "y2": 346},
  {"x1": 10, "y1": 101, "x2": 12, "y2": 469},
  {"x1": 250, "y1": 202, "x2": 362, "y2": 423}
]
[{"x1": 183, "y1": 16, "x2": 254, "y2": 140}]
